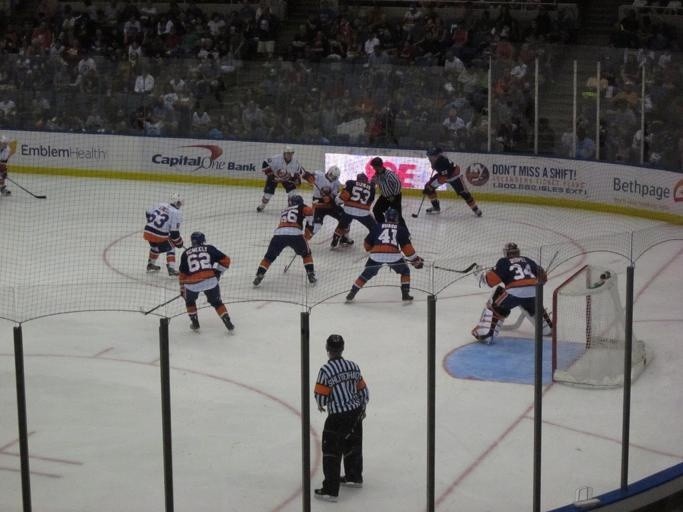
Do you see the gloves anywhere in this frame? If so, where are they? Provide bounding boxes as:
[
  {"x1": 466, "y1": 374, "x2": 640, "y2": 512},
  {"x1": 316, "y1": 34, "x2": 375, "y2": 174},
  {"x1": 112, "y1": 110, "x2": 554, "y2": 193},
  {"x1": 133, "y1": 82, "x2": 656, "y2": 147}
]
[
  {"x1": 181, "y1": 287, "x2": 184, "y2": 296},
  {"x1": 175, "y1": 237, "x2": 183, "y2": 248},
  {"x1": 304, "y1": 228, "x2": 312, "y2": 240},
  {"x1": 411, "y1": 256, "x2": 424, "y2": 269},
  {"x1": 306, "y1": 175, "x2": 315, "y2": 183}
]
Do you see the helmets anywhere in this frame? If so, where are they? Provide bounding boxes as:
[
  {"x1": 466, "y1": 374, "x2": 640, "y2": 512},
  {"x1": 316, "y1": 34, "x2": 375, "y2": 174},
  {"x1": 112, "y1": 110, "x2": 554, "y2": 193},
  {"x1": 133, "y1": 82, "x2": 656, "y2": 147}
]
[
  {"x1": 357, "y1": 174, "x2": 369, "y2": 183},
  {"x1": 292, "y1": 196, "x2": 303, "y2": 205},
  {"x1": 387, "y1": 208, "x2": 399, "y2": 222},
  {"x1": 1, "y1": 136, "x2": 9, "y2": 143},
  {"x1": 327, "y1": 165, "x2": 341, "y2": 184},
  {"x1": 426, "y1": 148, "x2": 438, "y2": 156},
  {"x1": 191, "y1": 232, "x2": 205, "y2": 244},
  {"x1": 170, "y1": 193, "x2": 184, "y2": 210},
  {"x1": 326, "y1": 335, "x2": 344, "y2": 352},
  {"x1": 370, "y1": 157, "x2": 382, "y2": 168},
  {"x1": 503, "y1": 242, "x2": 519, "y2": 257},
  {"x1": 284, "y1": 146, "x2": 295, "y2": 154}
]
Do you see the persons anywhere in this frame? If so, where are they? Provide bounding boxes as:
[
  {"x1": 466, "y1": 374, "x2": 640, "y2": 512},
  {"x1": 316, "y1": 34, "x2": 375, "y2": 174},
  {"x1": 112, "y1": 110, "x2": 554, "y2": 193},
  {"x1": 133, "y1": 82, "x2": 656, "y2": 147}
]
[
  {"x1": 253, "y1": 146, "x2": 424, "y2": 302},
  {"x1": 424, "y1": 147, "x2": 482, "y2": 217},
  {"x1": 144, "y1": 192, "x2": 185, "y2": 279},
  {"x1": 314, "y1": 334, "x2": 369, "y2": 498},
  {"x1": 2, "y1": 1, "x2": 682, "y2": 170},
  {"x1": 471, "y1": 241, "x2": 553, "y2": 343},
  {"x1": 178, "y1": 230, "x2": 235, "y2": 332},
  {"x1": 0, "y1": 139, "x2": 17, "y2": 195}
]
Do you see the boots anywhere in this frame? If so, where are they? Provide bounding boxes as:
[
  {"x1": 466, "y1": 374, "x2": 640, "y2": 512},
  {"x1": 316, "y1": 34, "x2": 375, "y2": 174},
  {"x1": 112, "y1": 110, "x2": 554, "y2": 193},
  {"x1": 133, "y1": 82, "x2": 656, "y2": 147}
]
[
  {"x1": 166, "y1": 264, "x2": 178, "y2": 275},
  {"x1": 426, "y1": 201, "x2": 440, "y2": 212},
  {"x1": 342, "y1": 235, "x2": 354, "y2": 244},
  {"x1": 473, "y1": 207, "x2": 482, "y2": 216},
  {"x1": 190, "y1": 319, "x2": 200, "y2": 329},
  {"x1": 402, "y1": 291, "x2": 413, "y2": 301},
  {"x1": 308, "y1": 272, "x2": 315, "y2": 282},
  {"x1": 254, "y1": 274, "x2": 264, "y2": 286},
  {"x1": 347, "y1": 289, "x2": 356, "y2": 300},
  {"x1": 1, "y1": 186, "x2": 11, "y2": 194},
  {"x1": 331, "y1": 232, "x2": 340, "y2": 247},
  {"x1": 223, "y1": 317, "x2": 233, "y2": 329},
  {"x1": 147, "y1": 259, "x2": 160, "y2": 270},
  {"x1": 257, "y1": 202, "x2": 266, "y2": 211}
]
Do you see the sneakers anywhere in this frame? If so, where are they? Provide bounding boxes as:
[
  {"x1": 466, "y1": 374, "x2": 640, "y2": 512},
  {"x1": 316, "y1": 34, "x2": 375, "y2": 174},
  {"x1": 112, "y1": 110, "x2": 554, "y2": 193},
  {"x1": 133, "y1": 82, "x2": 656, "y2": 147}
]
[
  {"x1": 340, "y1": 476, "x2": 362, "y2": 483},
  {"x1": 315, "y1": 489, "x2": 330, "y2": 495}
]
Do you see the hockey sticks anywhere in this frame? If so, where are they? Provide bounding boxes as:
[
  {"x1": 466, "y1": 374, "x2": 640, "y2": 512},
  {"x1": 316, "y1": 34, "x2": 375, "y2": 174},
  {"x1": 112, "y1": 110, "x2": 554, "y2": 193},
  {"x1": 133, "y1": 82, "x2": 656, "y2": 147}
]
[
  {"x1": 412, "y1": 170, "x2": 434, "y2": 218},
  {"x1": 140, "y1": 294, "x2": 181, "y2": 315},
  {"x1": 405, "y1": 259, "x2": 476, "y2": 273},
  {"x1": 284, "y1": 253, "x2": 297, "y2": 272},
  {"x1": 502, "y1": 251, "x2": 559, "y2": 331},
  {"x1": 6, "y1": 177, "x2": 47, "y2": 198}
]
